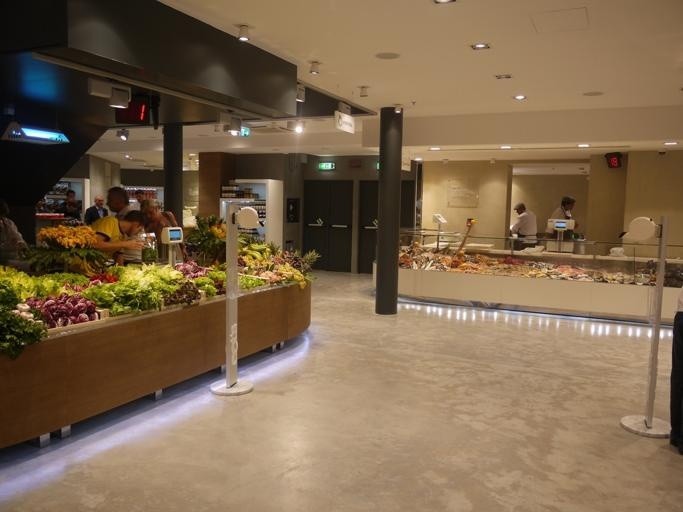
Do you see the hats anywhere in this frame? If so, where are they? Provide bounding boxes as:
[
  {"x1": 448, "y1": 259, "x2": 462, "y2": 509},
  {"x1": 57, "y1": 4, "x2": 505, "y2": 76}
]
[
  {"x1": 513, "y1": 203, "x2": 526, "y2": 210},
  {"x1": 562, "y1": 196, "x2": 576, "y2": 205}
]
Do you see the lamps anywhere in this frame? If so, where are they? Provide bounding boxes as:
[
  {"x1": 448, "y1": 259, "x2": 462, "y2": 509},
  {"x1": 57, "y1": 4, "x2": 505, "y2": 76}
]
[
  {"x1": 228, "y1": 117, "x2": 242, "y2": 137},
  {"x1": 117, "y1": 129, "x2": 129, "y2": 141},
  {"x1": 88, "y1": 77, "x2": 132, "y2": 109},
  {"x1": 1, "y1": 120, "x2": 70, "y2": 145},
  {"x1": 238, "y1": 24, "x2": 251, "y2": 42},
  {"x1": 358, "y1": 85, "x2": 370, "y2": 98},
  {"x1": 287, "y1": 120, "x2": 305, "y2": 134},
  {"x1": 309, "y1": 60, "x2": 322, "y2": 75}
]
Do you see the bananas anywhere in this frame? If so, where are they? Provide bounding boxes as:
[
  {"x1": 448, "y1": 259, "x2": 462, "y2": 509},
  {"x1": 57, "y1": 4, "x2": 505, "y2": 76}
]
[{"x1": 240, "y1": 242, "x2": 273, "y2": 259}]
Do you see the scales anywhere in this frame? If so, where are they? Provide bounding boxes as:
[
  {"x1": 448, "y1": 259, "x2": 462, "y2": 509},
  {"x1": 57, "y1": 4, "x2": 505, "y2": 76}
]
[
  {"x1": 162, "y1": 227, "x2": 184, "y2": 268},
  {"x1": 433, "y1": 214, "x2": 463, "y2": 243},
  {"x1": 546, "y1": 219, "x2": 575, "y2": 253}
]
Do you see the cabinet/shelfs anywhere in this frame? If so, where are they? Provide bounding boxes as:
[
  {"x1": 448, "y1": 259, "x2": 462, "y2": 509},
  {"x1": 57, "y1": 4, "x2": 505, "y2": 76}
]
[
  {"x1": 219, "y1": 178, "x2": 284, "y2": 254},
  {"x1": 46, "y1": 177, "x2": 91, "y2": 222}
]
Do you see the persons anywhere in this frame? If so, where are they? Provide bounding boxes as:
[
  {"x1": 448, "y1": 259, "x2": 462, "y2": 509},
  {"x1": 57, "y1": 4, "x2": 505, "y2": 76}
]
[
  {"x1": 85, "y1": 194, "x2": 108, "y2": 222},
  {"x1": 140, "y1": 199, "x2": 188, "y2": 262},
  {"x1": 546, "y1": 196, "x2": 578, "y2": 239},
  {"x1": 134, "y1": 190, "x2": 146, "y2": 202},
  {"x1": 86, "y1": 209, "x2": 145, "y2": 264},
  {"x1": 64, "y1": 190, "x2": 83, "y2": 222},
  {"x1": 510, "y1": 203, "x2": 539, "y2": 250},
  {"x1": 106, "y1": 186, "x2": 144, "y2": 263}
]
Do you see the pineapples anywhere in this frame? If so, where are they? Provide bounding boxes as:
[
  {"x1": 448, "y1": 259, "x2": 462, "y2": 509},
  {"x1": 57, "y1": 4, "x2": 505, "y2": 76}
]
[{"x1": 292, "y1": 248, "x2": 323, "y2": 273}]
